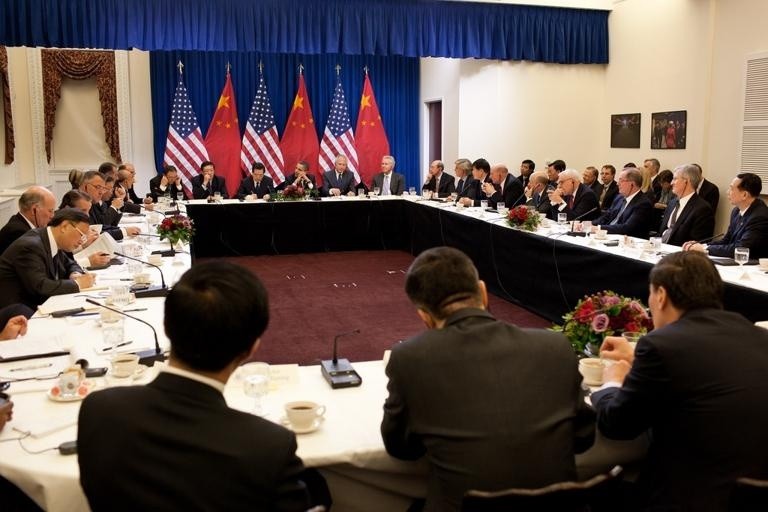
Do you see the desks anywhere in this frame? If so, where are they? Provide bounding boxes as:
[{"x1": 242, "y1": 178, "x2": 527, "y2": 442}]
[{"x1": 0, "y1": 193, "x2": 768, "y2": 512}]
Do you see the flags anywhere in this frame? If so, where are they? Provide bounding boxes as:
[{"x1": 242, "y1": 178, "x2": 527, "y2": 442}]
[
  {"x1": 318, "y1": 65, "x2": 361, "y2": 186},
  {"x1": 355, "y1": 65, "x2": 390, "y2": 191},
  {"x1": 162, "y1": 61, "x2": 210, "y2": 199},
  {"x1": 241, "y1": 61, "x2": 287, "y2": 189},
  {"x1": 280, "y1": 64, "x2": 320, "y2": 189},
  {"x1": 205, "y1": 61, "x2": 241, "y2": 198}
]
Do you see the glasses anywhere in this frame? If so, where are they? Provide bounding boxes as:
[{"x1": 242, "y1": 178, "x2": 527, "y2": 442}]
[
  {"x1": 87, "y1": 183, "x2": 106, "y2": 191},
  {"x1": 69, "y1": 221, "x2": 88, "y2": 243},
  {"x1": 559, "y1": 177, "x2": 571, "y2": 184},
  {"x1": 619, "y1": 178, "x2": 629, "y2": 183}
]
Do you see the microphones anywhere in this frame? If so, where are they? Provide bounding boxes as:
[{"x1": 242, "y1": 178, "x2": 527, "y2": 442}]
[
  {"x1": 453, "y1": 183, "x2": 471, "y2": 206},
  {"x1": 430, "y1": 178, "x2": 440, "y2": 201},
  {"x1": 202, "y1": 181, "x2": 216, "y2": 201},
  {"x1": 565, "y1": 208, "x2": 598, "y2": 237},
  {"x1": 302, "y1": 179, "x2": 310, "y2": 196},
  {"x1": 562, "y1": 308, "x2": 608, "y2": 336},
  {"x1": 509, "y1": 191, "x2": 527, "y2": 210},
  {"x1": 266, "y1": 183, "x2": 275, "y2": 201},
  {"x1": 689, "y1": 233, "x2": 724, "y2": 254},
  {"x1": 84, "y1": 298, "x2": 165, "y2": 367},
  {"x1": 130, "y1": 233, "x2": 176, "y2": 256},
  {"x1": 113, "y1": 251, "x2": 171, "y2": 296},
  {"x1": 128, "y1": 201, "x2": 167, "y2": 222}
]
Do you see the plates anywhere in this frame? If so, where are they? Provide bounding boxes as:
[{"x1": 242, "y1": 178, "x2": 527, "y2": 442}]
[
  {"x1": 105, "y1": 364, "x2": 148, "y2": 380},
  {"x1": 594, "y1": 237, "x2": 608, "y2": 240},
  {"x1": 48, "y1": 384, "x2": 91, "y2": 402},
  {"x1": 582, "y1": 380, "x2": 607, "y2": 386},
  {"x1": 758, "y1": 266, "x2": 768, "y2": 271},
  {"x1": 79, "y1": 379, "x2": 98, "y2": 389},
  {"x1": 282, "y1": 421, "x2": 326, "y2": 433}
]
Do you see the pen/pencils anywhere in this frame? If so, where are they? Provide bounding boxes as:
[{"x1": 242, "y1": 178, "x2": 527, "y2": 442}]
[
  {"x1": 100, "y1": 252, "x2": 110, "y2": 257},
  {"x1": 82, "y1": 266, "x2": 99, "y2": 286}
]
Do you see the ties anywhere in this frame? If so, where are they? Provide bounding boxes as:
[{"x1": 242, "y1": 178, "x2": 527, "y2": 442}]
[
  {"x1": 383, "y1": 176, "x2": 390, "y2": 196},
  {"x1": 435, "y1": 178, "x2": 439, "y2": 193},
  {"x1": 567, "y1": 196, "x2": 574, "y2": 209},
  {"x1": 611, "y1": 200, "x2": 628, "y2": 225},
  {"x1": 337, "y1": 174, "x2": 342, "y2": 185},
  {"x1": 598, "y1": 186, "x2": 608, "y2": 208},
  {"x1": 661, "y1": 202, "x2": 681, "y2": 242},
  {"x1": 207, "y1": 181, "x2": 211, "y2": 193},
  {"x1": 255, "y1": 182, "x2": 260, "y2": 191}
]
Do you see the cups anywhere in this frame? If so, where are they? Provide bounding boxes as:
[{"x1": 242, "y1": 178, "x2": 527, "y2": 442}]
[
  {"x1": 214, "y1": 192, "x2": 220, "y2": 202},
  {"x1": 358, "y1": 189, "x2": 364, "y2": 198},
  {"x1": 481, "y1": 200, "x2": 488, "y2": 213},
  {"x1": 557, "y1": 213, "x2": 567, "y2": 232},
  {"x1": 409, "y1": 187, "x2": 415, "y2": 196},
  {"x1": 423, "y1": 190, "x2": 428, "y2": 199},
  {"x1": 734, "y1": 248, "x2": 749, "y2": 270},
  {"x1": 177, "y1": 192, "x2": 183, "y2": 201},
  {"x1": 582, "y1": 221, "x2": 592, "y2": 241},
  {"x1": 58, "y1": 373, "x2": 78, "y2": 398},
  {"x1": 285, "y1": 401, "x2": 325, "y2": 426},
  {"x1": 451, "y1": 193, "x2": 458, "y2": 204},
  {"x1": 649, "y1": 237, "x2": 662, "y2": 255},
  {"x1": 63, "y1": 363, "x2": 85, "y2": 386},
  {"x1": 110, "y1": 353, "x2": 140, "y2": 376},
  {"x1": 277, "y1": 190, "x2": 283, "y2": 198},
  {"x1": 497, "y1": 203, "x2": 505, "y2": 219},
  {"x1": 594, "y1": 230, "x2": 608, "y2": 237},
  {"x1": 239, "y1": 361, "x2": 273, "y2": 419},
  {"x1": 578, "y1": 357, "x2": 612, "y2": 382},
  {"x1": 759, "y1": 258, "x2": 768, "y2": 268},
  {"x1": 623, "y1": 331, "x2": 646, "y2": 353},
  {"x1": 374, "y1": 187, "x2": 380, "y2": 197}
]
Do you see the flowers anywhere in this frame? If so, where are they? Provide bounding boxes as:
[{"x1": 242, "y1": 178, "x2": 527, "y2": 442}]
[
  {"x1": 155, "y1": 215, "x2": 196, "y2": 245},
  {"x1": 271, "y1": 184, "x2": 312, "y2": 201},
  {"x1": 503, "y1": 204, "x2": 544, "y2": 232},
  {"x1": 544, "y1": 289, "x2": 654, "y2": 358}
]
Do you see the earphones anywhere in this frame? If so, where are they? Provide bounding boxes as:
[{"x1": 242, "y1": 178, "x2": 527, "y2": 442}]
[
  {"x1": 320, "y1": 329, "x2": 362, "y2": 389},
  {"x1": 156, "y1": 193, "x2": 180, "y2": 215},
  {"x1": 534, "y1": 183, "x2": 540, "y2": 190},
  {"x1": 82, "y1": 183, "x2": 87, "y2": 192},
  {"x1": 498, "y1": 172, "x2": 502, "y2": 182},
  {"x1": 32, "y1": 207, "x2": 39, "y2": 231},
  {"x1": 460, "y1": 169, "x2": 465, "y2": 181},
  {"x1": 571, "y1": 180, "x2": 575, "y2": 193},
  {"x1": 626, "y1": 183, "x2": 633, "y2": 198},
  {"x1": 680, "y1": 181, "x2": 687, "y2": 198}
]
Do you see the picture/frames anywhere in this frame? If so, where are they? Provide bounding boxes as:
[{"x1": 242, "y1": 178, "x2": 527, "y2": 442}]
[
  {"x1": 651, "y1": 110, "x2": 687, "y2": 150},
  {"x1": 610, "y1": 113, "x2": 642, "y2": 149}
]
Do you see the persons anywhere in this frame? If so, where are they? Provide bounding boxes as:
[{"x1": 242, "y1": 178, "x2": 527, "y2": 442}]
[
  {"x1": 118, "y1": 164, "x2": 152, "y2": 205},
  {"x1": 481, "y1": 164, "x2": 527, "y2": 210},
  {"x1": 98, "y1": 162, "x2": 119, "y2": 186},
  {"x1": 94, "y1": 177, "x2": 126, "y2": 227},
  {"x1": 517, "y1": 159, "x2": 535, "y2": 189},
  {"x1": 592, "y1": 167, "x2": 652, "y2": 240},
  {"x1": 599, "y1": 165, "x2": 619, "y2": 216},
  {"x1": 79, "y1": 171, "x2": 141, "y2": 240},
  {"x1": 422, "y1": 160, "x2": 455, "y2": 198},
  {"x1": 643, "y1": 159, "x2": 662, "y2": 198},
  {"x1": 624, "y1": 163, "x2": 637, "y2": 168},
  {"x1": 548, "y1": 163, "x2": 562, "y2": 189},
  {"x1": 0, "y1": 314, "x2": 28, "y2": 340},
  {"x1": 54, "y1": 189, "x2": 99, "y2": 255},
  {"x1": 0, "y1": 185, "x2": 110, "y2": 268},
  {"x1": 320, "y1": 155, "x2": 355, "y2": 196},
  {"x1": 283, "y1": 161, "x2": 316, "y2": 197},
  {"x1": 583, "y1": 166, "x2": 603, "y2": 201},
  {"x1": 650, "y1": 170, "x2": 677, "y2": 241},
  {"x1": 237, "y1": 162, "x2": 274, "y2": 200},
  {"x1": 448, "y1": 159, "x2": 473, "y2": 201},
  {"x1": 654, "y1": 165, "x2": 715, "y2": 247},
  {"x1": 688, "y1": 164, "x2": 719, "y2": 215},
  {"x1": 682, "y1": 173, "x2": 768, "y2": 260},
  {"x1": 150, "y1": 166, "x2": 185, "y2": 203},
  {"x1": 370, "y1": 156, "x2": 405, "y2": 196},
  {"x1": 524, "y1": 171, "x2": 555, "y2": 220},
  {"x1": 381, "y1": 248, "x2": 598, "y2": 512},
  {"x1": 591, "y1": 251, "x2": 768, "y2": 512},
  {"x1": 0, "y1": 208, "x2": 96, "y2": 312},
  {"x1": 635, "y1": 166, "x2": 655, "y2": 208},
  {"x1": 0, "y1": 396, "x2": 14, "y2": 434},
  {"x1": 554, "y1": 160, "x2": 566, "y2": 171},
  {"x1": 76, "y1": 260, "x2": 333, "y2": 512},
  {"x1": 547, "y1": 169, "x2": 601, "y2": 225},
  {"x1": 192, "y1": 161, "x2": 229, "y2": 199},
  {"x1": 456, "y1": 159, "x2": 498, "y2": 207},
  {"x1": 112, "y1": 170, "x2": 154, "y2": 214}
]
